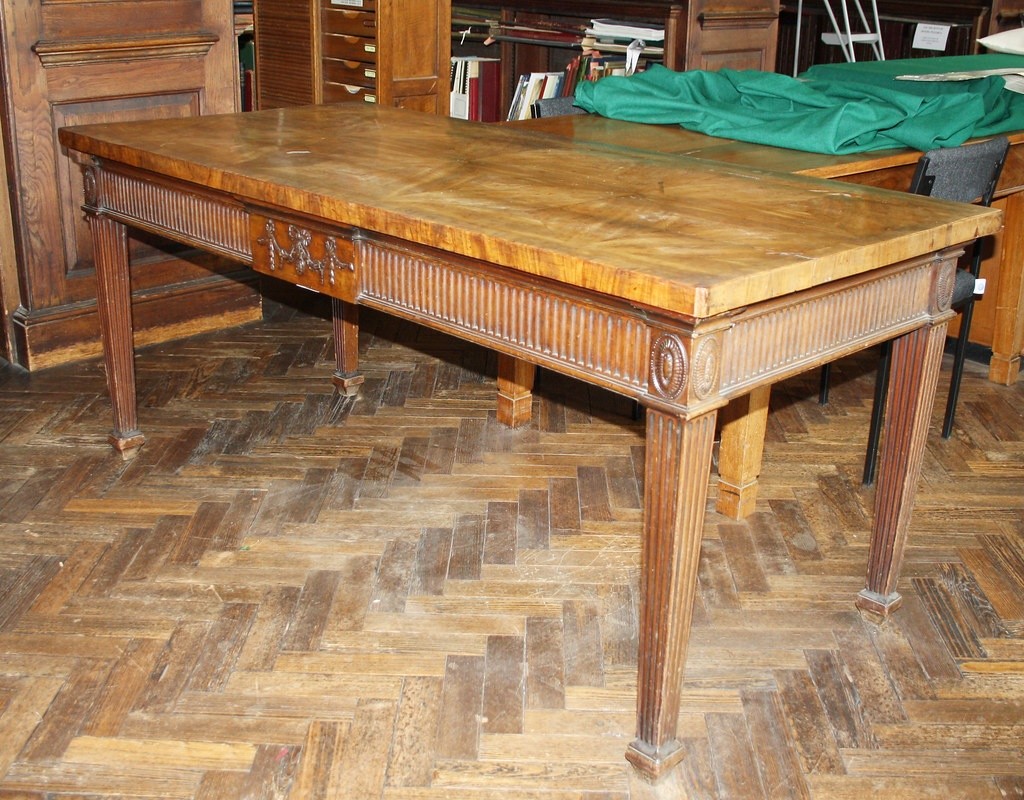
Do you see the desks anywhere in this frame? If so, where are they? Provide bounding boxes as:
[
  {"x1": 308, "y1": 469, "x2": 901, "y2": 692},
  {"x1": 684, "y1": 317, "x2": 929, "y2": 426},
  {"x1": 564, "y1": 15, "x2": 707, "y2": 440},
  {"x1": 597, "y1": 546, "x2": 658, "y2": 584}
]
[
  {"x1": 495, "y1": 53, "x2": 1023, "y2": 522},
  {"x1": 58, "y1": 100, "x2": 1003, "y2": 787}
]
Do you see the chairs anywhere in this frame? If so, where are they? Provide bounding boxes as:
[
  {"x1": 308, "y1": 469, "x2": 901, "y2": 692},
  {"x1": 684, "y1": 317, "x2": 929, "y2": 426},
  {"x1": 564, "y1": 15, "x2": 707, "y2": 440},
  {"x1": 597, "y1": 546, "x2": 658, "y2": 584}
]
[{"x1": 818, "y1": 134, "x2": 1012, "y2": 486}]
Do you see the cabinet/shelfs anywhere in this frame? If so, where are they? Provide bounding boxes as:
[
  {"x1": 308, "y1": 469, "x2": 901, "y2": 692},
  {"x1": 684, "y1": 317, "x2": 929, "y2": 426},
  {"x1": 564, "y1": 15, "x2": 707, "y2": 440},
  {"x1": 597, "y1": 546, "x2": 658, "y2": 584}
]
[
  {"x1": 774, "y1": 0, "x2": 1024, "y2": 78},
  {"x1": 253, "y1": 0, "x2": 452, "y2": 117},
  {"x1": 450, "y1": 0, "x2": 779, "y2": 123},
  {"x1": 0, "y1": 0, "x2": 265, "y2": 374}
]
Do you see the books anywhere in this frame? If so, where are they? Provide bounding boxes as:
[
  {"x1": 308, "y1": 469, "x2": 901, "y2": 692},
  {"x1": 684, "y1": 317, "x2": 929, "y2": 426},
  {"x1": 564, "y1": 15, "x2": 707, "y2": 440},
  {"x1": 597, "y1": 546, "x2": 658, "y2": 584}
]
[
  {"x1": 777, "y1": 17, "x2": 971, "y2": 78},
  {"x1": 451, "y1": 55, "x2": 501, "y2": 123},
  {"x1": 505, "y1": 55, "x2": 663, "y2": 121},
  {"x1": 451, "y1": 5, "x2": 665, "y2": 54}
]
